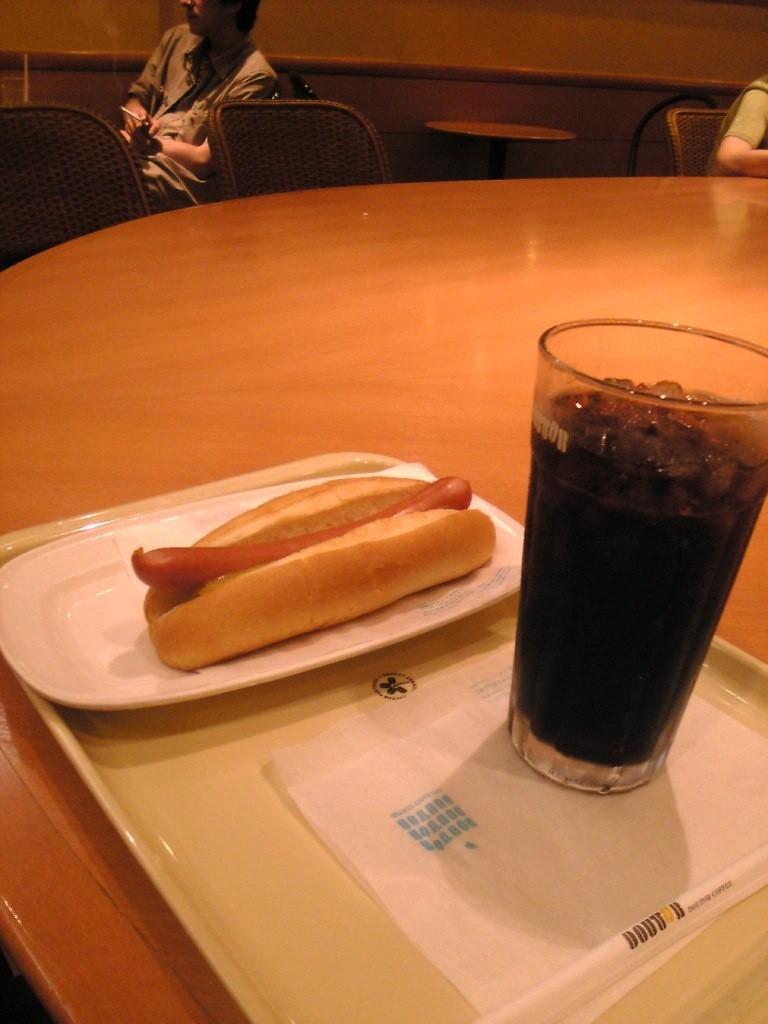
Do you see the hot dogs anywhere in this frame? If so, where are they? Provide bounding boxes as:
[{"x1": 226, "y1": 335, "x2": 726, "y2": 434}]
[{"x1": 131, "y1": 474, "x2": 496, "y2": 674}]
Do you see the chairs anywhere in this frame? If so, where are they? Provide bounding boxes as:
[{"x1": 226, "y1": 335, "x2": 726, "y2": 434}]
[
  {"x1": 206, "y1": 97, "x2": 394, "y2": 199},
  {"x1": 0, "y1": 101, "x2": 150, "y2": 277},
  {"x1": 666, "y1": 106, "x2": 728, "y2": 177}
]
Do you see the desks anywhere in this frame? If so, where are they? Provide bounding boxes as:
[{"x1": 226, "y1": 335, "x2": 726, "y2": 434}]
[{"x1": 423, "y1": 120, "x2": 576, "y2": 180}]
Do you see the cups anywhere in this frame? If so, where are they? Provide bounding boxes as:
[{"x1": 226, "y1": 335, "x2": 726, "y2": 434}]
[{"x1": 507, "y1": 321, "x2": 767, "y2": 795}]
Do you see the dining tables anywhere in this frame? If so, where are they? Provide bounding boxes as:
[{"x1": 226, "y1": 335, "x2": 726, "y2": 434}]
[{"x1": 0, "y1": 179, "x2": 768, "y2": 1024}]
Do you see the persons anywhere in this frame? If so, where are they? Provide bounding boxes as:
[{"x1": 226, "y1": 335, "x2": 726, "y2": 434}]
[
  {"x1": 118, "y1": 0, "x2": 277, "y2": 211},
  {"x1": 707, "y1": 73, "x2": 768, "y2": 178}
]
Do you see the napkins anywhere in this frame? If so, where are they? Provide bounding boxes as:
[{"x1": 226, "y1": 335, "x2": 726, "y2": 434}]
[{"x1": 272, "y1": 642, "x2": 768, "y2": 1024}]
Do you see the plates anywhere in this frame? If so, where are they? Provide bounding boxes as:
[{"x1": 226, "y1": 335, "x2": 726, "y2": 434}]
[{"x1": 1, "y1": 472, "x2": 522, "y2": 711}]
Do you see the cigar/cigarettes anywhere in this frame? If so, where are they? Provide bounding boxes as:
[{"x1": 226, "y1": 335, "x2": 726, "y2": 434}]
[{"x1": 120, "y1": 107, "x2": 149, "y2": 126}]
